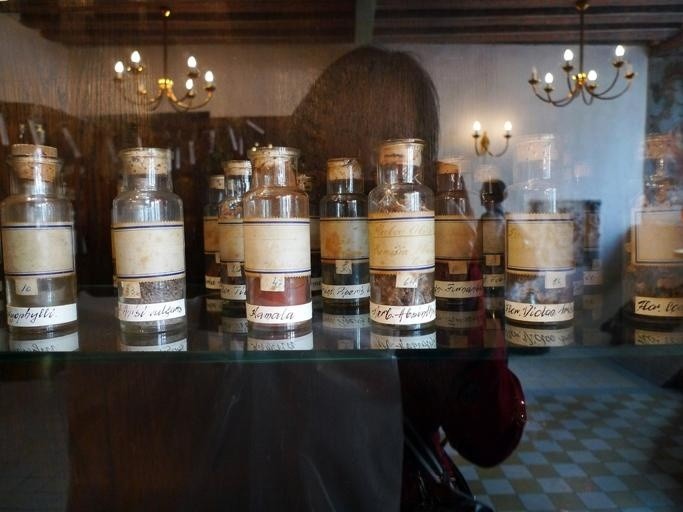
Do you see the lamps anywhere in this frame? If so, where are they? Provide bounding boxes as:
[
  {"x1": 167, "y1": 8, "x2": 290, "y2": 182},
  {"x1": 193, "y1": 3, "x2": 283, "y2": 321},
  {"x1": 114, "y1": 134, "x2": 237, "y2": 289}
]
[
  {"x1": 528, "y1": 3, "x2": 635, "y2": 107},
  {"x1": 114, "y1": 7, "x2": 215, "y2": 113},
  {"x1": 472, "y1": 119, "x2": 511, "y2": 157}
]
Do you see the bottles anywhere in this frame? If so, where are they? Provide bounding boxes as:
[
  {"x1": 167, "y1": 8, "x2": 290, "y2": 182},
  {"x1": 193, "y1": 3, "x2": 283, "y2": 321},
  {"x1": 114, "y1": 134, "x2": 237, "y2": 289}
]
[{"x1": 0, "y1": 134, "x2": 681, "y2": 355}]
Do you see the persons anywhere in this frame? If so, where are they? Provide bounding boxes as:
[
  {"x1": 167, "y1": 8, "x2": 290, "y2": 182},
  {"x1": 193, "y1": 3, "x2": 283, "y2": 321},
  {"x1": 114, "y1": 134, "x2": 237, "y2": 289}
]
[{"x1": 68, "y1": 44, "x2": 526, "y2": 506}]
